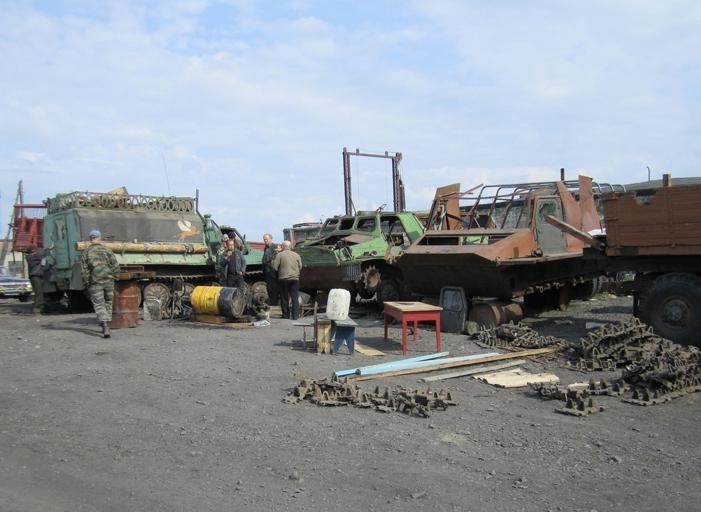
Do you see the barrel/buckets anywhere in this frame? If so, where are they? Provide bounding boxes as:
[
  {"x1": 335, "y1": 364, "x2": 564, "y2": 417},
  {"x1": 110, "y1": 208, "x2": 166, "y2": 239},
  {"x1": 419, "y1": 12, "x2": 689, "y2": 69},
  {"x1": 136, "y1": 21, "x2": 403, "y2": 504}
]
[
  {"x1": 468, "y1": 300, "x2": 524, "y2": 331},
  {"x1": 107, "y1": 280, "x2": 141, "y2": 329},
  {"x1": 325, "y1": 287, "x2": 351, "y2": 320},
  {"x1": 191, "y1": 286, "x2": 246, "y2": 318}
]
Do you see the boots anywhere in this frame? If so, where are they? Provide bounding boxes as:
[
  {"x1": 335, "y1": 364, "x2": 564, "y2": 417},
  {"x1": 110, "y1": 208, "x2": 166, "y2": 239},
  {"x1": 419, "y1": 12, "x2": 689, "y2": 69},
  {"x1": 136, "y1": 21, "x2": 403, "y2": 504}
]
[{"x1": 101, "y1": 322, "x2": 109, "y2": 337}]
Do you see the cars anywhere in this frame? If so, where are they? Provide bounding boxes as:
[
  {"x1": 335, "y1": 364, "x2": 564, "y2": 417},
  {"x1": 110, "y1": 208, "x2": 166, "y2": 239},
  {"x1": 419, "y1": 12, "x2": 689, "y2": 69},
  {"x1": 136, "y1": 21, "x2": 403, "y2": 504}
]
[{"x1": 0, "y1": 266, "x2": 32, "y2": 302}]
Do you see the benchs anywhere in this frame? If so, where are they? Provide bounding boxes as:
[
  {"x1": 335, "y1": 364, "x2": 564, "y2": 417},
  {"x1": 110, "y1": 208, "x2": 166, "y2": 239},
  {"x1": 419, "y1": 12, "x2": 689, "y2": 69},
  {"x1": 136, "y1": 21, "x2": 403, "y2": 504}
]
[{"x1": 289, "y1": 313, "x2": 359, "y2": 355}]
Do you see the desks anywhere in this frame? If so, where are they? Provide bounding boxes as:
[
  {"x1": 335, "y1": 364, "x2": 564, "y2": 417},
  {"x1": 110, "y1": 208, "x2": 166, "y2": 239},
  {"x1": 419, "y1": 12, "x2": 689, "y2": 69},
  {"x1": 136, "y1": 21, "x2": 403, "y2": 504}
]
[{"x1": 382, "y1": 301, "x2": 443, "y2": 353}]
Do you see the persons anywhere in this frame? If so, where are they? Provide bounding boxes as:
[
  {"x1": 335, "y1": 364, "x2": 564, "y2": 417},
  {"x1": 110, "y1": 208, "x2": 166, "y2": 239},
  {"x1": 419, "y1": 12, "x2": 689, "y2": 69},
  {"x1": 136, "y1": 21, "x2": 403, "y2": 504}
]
[
  {"x1": 228, "y1": 232, "x2": 243, "y2": 251},
  {"x1": 78, "y1": 229, "x2": 121, "y2": 338},
  {"x1": 219, "y1": 240, "x2": 247, "y2": 288},
  {"x1": 212, "y1": 234, "x2": 229, "y2": 286},
  {"x1": 22, "y1": 240, "x2": 56, "y2": 316},
  {"x1": 261, "y1": 233, "x2": 279, "y2": 306},
  {"x1": 269, "y1": 240, "x2": 303, "y2": 320}
]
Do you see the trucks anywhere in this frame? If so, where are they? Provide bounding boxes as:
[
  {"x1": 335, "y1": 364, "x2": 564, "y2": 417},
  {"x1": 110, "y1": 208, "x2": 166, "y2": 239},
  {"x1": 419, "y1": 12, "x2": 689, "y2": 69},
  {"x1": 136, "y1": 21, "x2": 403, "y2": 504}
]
[{"x1": 33, "y1": 193, "x2": 267, "y2": 315}]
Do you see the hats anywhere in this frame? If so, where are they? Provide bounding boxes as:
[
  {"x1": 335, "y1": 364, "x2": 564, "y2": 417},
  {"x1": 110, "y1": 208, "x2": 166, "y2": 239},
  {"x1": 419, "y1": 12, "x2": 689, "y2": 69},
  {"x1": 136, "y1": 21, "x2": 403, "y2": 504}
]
[
  {"x1": 228, "y1": 232, "x2": 234, "y2": 237},
  {"x1": 24, "y1": 245, "x2": 37, "y2": 254},
  {"x1": 222, "y1": 234, "x2": 229, "y2": 241},
  {"x1": 89, "y1": 230, "x2": 102, "y2": 238}
]
